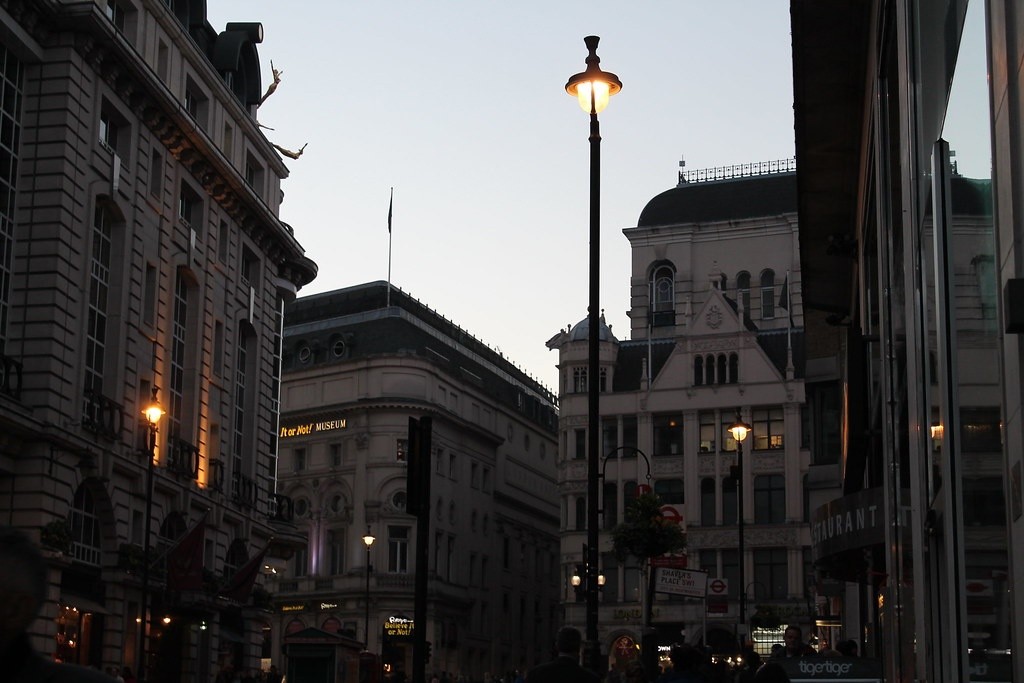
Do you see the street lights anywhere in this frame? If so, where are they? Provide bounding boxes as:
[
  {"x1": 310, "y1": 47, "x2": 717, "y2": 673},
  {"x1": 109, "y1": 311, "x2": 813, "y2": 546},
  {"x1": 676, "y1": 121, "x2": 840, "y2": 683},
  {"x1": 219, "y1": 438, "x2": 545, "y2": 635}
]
[
  {"x1": 563, "y1": 33, "x2": 651, "y2": 683},
  {"x1": 726, "y1": 415, "x2": 765, "y2": 640},
  {"x1": 362, "y1": 522, "x2": 377, "y2": 648},
  {"x1": 138, "y1": 384, "x2": 166, "y2": 683}
]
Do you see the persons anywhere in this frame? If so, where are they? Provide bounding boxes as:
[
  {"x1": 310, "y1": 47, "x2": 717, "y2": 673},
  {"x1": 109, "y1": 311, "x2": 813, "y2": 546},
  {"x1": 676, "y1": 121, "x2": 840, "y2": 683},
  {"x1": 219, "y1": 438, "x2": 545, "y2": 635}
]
[
  {"x1": 524, "y1": 625, "x2": 599, "y2": 683},
  {"x1": 236, "y1": 664, "x2": 282, "y2": 683},
  {"x1": 0, "y1": 528, "x2": 125, "y2": 683},
  {"x1": 104, "y1": 662, "x2": 139, "y2": 683},
  {"x1": 822, "y1": 636, "x2": 860, "y2": 661},
  {"x1": 769, "y1": 625, "x2": 819, "y2": 659},
  {"x1": 605, "y1": 659, "x2": 653, "y2": 683},
  {"x1": 656, "y1": 638, "x2": 794, "y2": 683},
  {"x1": 430, "y1": 666, "x2": 524, "y2": 683}
]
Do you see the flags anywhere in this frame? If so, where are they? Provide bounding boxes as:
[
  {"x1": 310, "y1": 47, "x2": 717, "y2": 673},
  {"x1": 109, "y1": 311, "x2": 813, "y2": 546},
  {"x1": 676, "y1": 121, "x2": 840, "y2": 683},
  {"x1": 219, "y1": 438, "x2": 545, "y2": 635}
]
[
  {"x1": 228, "y1": 543, "x2": 268, "y2": 600},
  {"x1": 159, "y1": 516, "x2": 205, "y2": 594}
]
